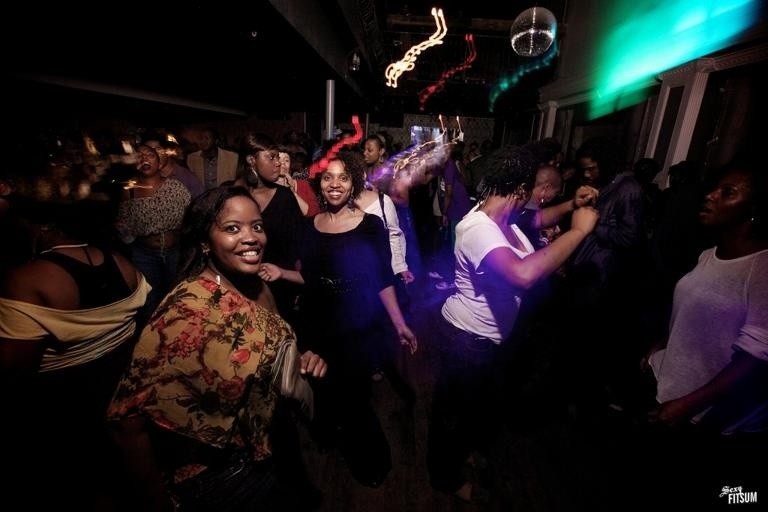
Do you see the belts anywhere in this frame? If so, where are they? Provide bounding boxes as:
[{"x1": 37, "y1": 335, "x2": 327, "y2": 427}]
[{"x1": 319, "y1": 276, "x2": 354, "y2": 295}]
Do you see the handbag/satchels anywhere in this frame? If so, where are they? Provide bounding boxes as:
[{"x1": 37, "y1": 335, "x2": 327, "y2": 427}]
[{"x1": 271, "y1": 331, "x2": 316, "y2": 419}]
